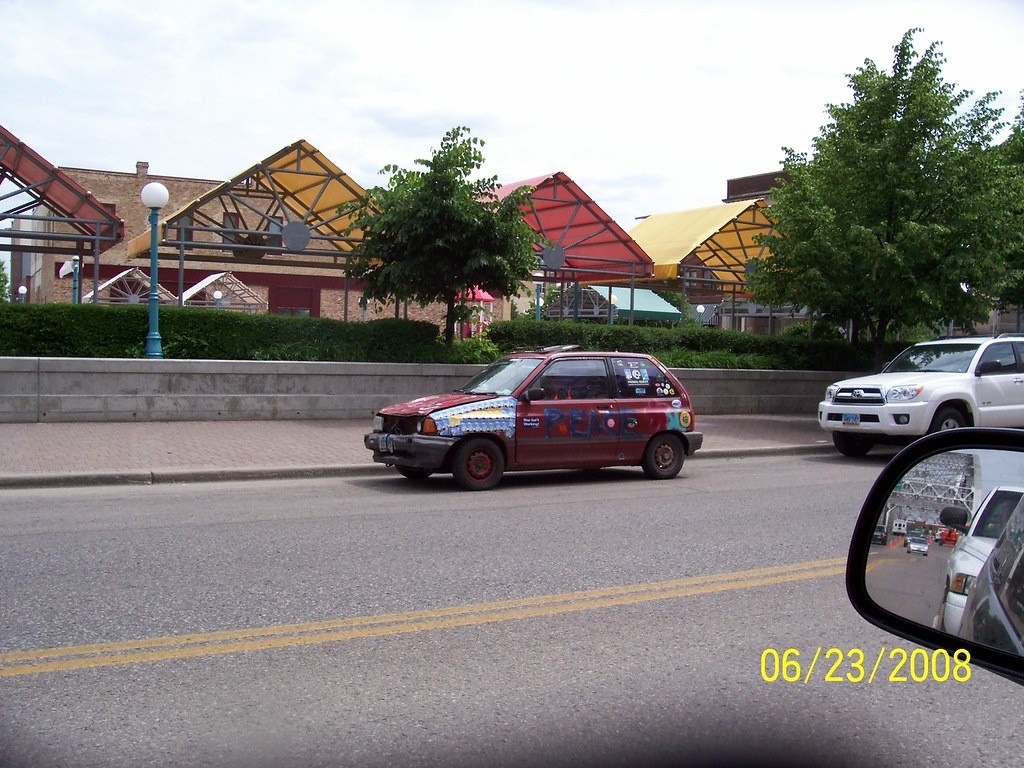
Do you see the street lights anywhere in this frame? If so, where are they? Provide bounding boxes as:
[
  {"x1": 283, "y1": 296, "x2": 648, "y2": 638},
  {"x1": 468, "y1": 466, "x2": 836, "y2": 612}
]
[
  {"x1": 72, "y1": 254, "x2": 80, "y2": 306},
  {"x1": 140, "y1": 180, "x2": 171, "y2": 359},
  {"x1": 17, "y1": 285, "x2": 27, "y2": 304}
]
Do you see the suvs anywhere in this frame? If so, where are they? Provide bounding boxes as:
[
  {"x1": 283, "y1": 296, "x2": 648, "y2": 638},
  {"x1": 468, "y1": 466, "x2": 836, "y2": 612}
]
[{"x1": 815, "y1": 332, "x2": 1024, "y2": 457}]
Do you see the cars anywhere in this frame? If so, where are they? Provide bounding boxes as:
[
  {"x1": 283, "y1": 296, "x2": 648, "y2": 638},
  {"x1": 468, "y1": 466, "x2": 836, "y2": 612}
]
[
  {"x1": 362, "y1": 346, "x2": 703, "y2": 493},
  {"x1": 870, "y1": 519, "x2": 957, "y2": 559},
  {"x1": 956, "y1": 494, "x2": 1024, "y2": 656},
  {"x1": 936, "y1": 485, "x2": 1023, "y2": 651}
]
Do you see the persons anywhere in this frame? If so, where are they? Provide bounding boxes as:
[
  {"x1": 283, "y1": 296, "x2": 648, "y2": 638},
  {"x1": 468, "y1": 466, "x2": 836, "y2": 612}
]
[{"x1": 965, "y1": 502, "x2": 1013, "y2": 529}]
